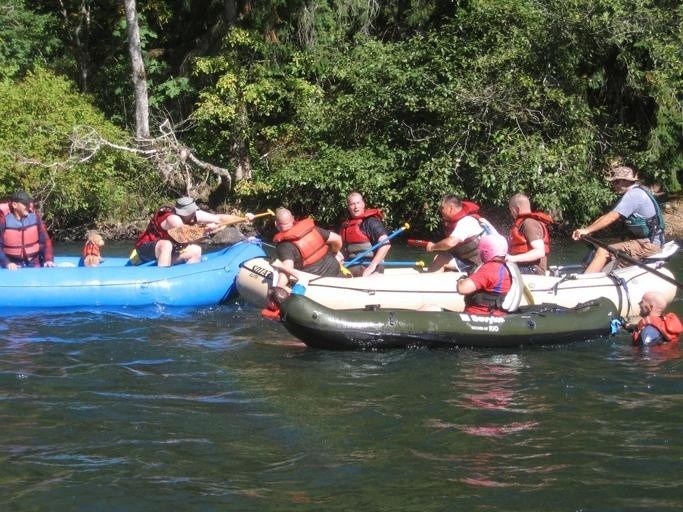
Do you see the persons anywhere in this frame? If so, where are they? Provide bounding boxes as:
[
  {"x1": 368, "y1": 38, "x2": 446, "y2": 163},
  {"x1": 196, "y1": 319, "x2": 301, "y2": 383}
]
[
  {"x1": 572, "y1": 166, "x2": 666, "y2": 273},
  {"x1": 505, "y1": 193, "x2": 555, "y2": 274},
  {"x1": 456, "y1": 233, "x2": 524, "y2": 316},
  {"x1": 134, "y1": 197, "x2": 255, "y2": 266},
  {"x1": 425, "y1": 193, "x2": 500, "y2": 273},
  {"x1": 620, "y1": 291, "x2": 683, "y2": 353},
  {"x1": 270, "y1": 208, "x2": 343, "y2": 287},
  {"x1": 338, "y1": 191, "x2": 392, "y2": 276},
  {"x1": 0, "y1": 192, "x2": 56, "y2": 272},
  {"x1": 261, "y1": 259, "x2": 309, "y2": 324}
]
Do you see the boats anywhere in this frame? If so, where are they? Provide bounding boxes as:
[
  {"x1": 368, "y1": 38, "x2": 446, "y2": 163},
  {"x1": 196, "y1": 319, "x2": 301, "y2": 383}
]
[
  {"x1": 271, "y1": 292, "x2": 626, "y2": 353},
  {"x1": 0, "y1": 235, "x2": 270, "y2": 308},
  {"x1": 231, "y1": 258, "x2": 679, "y2": 325},
  {"x1": 0, "y1": 308, "x2": 263, "y2": 366}
]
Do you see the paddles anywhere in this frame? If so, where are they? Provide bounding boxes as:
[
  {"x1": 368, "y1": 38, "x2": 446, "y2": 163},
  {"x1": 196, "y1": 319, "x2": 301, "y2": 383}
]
[
  {"x1": 168, "y1": 209, "x2": 275, "y2": 244},
  {"x1": 601, "y1": 259, "x2": 621, "y2": 274},
  {"x1": 523, "y1": 282, "x2": 534, "y2": 305}
]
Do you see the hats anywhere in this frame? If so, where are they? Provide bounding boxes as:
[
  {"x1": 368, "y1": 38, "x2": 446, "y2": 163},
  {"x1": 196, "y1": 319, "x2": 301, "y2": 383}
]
[
  {"x1": 11, "y1": 192, "x2": 31, "y2": 204},
  {"x1": 605, "y1": 167, "x2": 636, "y2": 181},
  {"x1": 175, "y1": 197, "x2": 199, "y2": 216}
]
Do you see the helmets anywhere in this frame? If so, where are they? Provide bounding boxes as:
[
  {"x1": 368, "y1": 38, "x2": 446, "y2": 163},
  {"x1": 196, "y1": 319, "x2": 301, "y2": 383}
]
[{"x1": 480, "y1": 234, "x2": 508, "y2": 263}]
